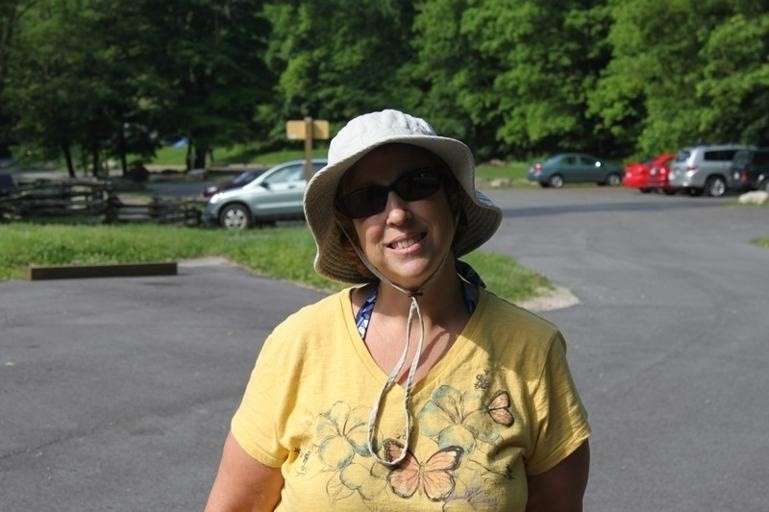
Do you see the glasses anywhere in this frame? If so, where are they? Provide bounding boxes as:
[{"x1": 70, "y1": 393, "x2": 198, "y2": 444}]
[{"x1": 336, "y1": 164, "x2": 444, "y2": 221}]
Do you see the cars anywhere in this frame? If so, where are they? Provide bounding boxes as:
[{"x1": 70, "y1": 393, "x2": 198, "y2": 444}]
[
  {"x1": 527, "y1": 151, "x2": 624, "y2": 189},
  {"x1": 202, "y1": 154, "x2": 328, "y2": 233},
  {"x1": 623, "y1": 142, "x2": 769, "y2": 198}
]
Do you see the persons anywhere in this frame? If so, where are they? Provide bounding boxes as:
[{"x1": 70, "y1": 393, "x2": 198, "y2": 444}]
[
  {"x1": 123, "y1": 159, "x2": 151, "y2": 181},
  {"x1": 204, "y1": 108, "x2": 593, "y2": 511}
]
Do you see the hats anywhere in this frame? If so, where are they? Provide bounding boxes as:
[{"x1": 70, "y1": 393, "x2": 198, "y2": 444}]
[{"x1": 303, "y1": 108, "x2": 504, "y2": 285}]
